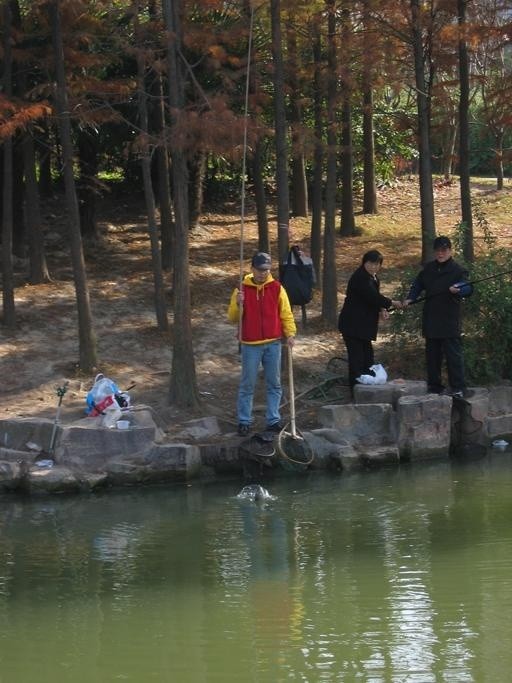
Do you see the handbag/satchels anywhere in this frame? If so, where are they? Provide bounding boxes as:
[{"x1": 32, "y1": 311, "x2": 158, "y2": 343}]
[{"x1": 279, "y1": 264, "x2": 312, "y2": 305}]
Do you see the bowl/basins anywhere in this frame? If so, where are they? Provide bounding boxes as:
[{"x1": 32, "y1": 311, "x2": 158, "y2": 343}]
[{"x1": 115, "y1": 420, "x2": 130, "y2": 430}]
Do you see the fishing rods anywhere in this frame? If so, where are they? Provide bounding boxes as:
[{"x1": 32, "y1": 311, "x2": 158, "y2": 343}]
[{"x1": 385, "y1": 271, "x2": 511, "y2": 313}]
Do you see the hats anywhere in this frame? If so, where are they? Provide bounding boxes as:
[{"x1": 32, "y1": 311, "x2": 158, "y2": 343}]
[
  {"x1": 434, "y1": 237, "x2": 451, "y2": 248},
  {"x1": 252, "y1": 252, "x2": 271, "y2": 270}
]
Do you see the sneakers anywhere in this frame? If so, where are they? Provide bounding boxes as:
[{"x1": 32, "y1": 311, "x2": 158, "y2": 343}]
[
  {"x1": 267, "y1": 422, "x2": 286, "y2": 432},
  {"x1": 452, "y1": 389, "x2": 464, "y2": 398},
  {"x1": 238, "y1": 424, "x2": 249, "y2": 435}
]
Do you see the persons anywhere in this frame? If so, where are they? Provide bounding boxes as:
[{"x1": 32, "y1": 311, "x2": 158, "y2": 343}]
[
  {"x1": 401, "y1": 232, "x2": 474, "y2": 395},
  {"x1": 225, "y1": 251, "x2": 297, "y2": 436},
  {"x1": 337, "y1": 247, "x2": 403, "y2": 400}
]
